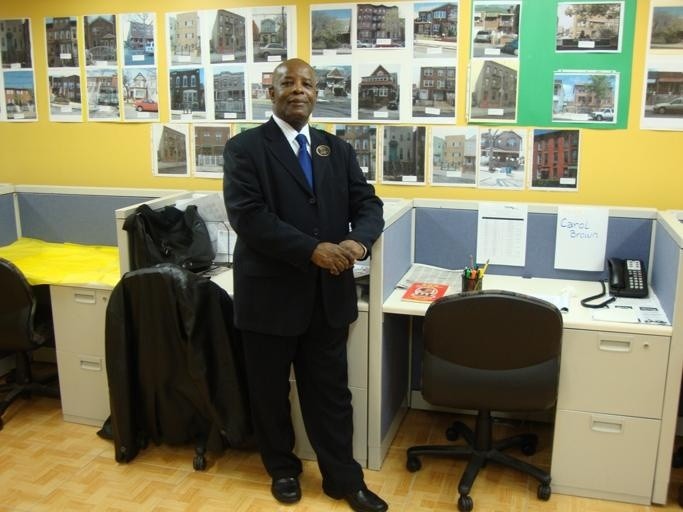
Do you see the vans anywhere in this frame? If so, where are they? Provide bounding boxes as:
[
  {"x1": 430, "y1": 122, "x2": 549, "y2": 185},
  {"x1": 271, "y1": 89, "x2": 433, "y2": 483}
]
[{"x1": 145, "y1": 41, "x2": 153, "y2": 54}]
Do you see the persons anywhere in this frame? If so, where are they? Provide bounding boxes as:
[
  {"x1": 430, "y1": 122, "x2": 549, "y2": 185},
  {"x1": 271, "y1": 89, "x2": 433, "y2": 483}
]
[{"x1": 222, "y1": 57, "x2": 389, "y2": 512}]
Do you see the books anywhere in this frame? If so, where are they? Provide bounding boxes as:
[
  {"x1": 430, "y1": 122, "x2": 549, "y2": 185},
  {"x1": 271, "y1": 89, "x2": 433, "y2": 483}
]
[{"x1": 401, "y1": 281, "x2": 451, "y2": 303}]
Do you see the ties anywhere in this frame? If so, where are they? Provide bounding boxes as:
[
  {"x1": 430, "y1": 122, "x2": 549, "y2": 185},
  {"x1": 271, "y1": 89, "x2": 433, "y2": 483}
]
[{"x1": 295, "y1": 133, "x2": 314, "y2": 193}]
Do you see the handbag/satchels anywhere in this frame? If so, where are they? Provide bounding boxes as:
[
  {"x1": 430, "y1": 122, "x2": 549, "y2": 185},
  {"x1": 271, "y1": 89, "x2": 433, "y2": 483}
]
[{"x1": 121, "y1": 204, "x2": 215, "y2": 274}]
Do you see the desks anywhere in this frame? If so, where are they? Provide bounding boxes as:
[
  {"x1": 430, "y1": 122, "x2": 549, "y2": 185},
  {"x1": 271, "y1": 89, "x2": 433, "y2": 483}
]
[
  {"x1": 369, "y1": 197, "x2": 683, "y2": 505},
  {"x1": 1, "y1": 183, "x2": 192, "y2": 429},
  {"x1": 114, "y1": 190, "x2": 368, "y2": 471}
]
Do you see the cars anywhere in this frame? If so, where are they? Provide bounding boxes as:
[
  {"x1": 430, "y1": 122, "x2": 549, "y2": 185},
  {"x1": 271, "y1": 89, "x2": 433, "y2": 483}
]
[
  {"x1": 591, "y1": 108, "x2": 614, "y2": 121},
  {"x1": 504, "y1": 37, "x2": 518, "y2": 56},
  {"x1": 259, "y1": 43, "x2": 287, "y2": 57},
  {"x1": 357, "y1": 40, "x2": 372, "y2": 48},
  {"x1": 134, "y1": 98, "x2": 158, "y2": 112},
  {"x1": 653, "y1": 97, "x2": 682, "y2": 115},
  {"x1": 99, "y1": 96, "x2": 118, "y2": 106}
]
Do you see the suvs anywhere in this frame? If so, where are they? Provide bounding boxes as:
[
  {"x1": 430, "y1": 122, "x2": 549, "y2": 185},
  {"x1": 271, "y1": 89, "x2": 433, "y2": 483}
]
[{"x1": 477, "y1": 30, "x2": 491, "y2": 43}]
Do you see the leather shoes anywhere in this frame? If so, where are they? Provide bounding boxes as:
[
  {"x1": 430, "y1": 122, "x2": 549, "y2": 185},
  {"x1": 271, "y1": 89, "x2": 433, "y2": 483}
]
[
  {"x1": 271, "y1": 476, "x2": 301, "y2": 503},
  {"x1": 344, "y1": 487, "x2": 387, "y2": 512}
]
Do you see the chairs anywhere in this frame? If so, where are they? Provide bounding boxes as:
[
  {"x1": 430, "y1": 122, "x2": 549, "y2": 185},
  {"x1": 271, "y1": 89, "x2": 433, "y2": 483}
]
[
  {"x1": 405, "y1": 289, "x2": 563, "y2": 512},
  {"x1": 96, "y1": 266, "x2": 247, "y2": 470},
  {"x1": 0, "y1": 258, "x2": 60, "y2": 431}
]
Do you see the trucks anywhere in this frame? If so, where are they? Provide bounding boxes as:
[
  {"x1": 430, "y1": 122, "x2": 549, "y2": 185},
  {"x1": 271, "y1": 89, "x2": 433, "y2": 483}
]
[{"x1": 375, "y1": 38, "x2": 402, "y2": 47}]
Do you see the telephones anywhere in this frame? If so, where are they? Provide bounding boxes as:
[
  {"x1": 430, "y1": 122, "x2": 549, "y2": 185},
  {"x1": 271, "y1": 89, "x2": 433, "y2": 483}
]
[{"x1": 608, "y1": 257, "x2": 648, "y2": 298}]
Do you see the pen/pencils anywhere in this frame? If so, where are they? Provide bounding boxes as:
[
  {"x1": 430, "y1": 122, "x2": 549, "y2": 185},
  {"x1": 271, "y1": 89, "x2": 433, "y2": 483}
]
[{"x1": 462, "y1": 255, "x2": 489, "y2": 291}]
